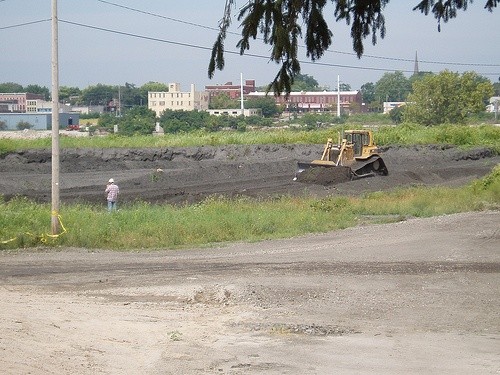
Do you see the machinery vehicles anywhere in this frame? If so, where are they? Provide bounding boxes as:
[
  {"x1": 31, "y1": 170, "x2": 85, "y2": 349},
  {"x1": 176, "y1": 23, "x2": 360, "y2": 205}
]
[{"x1": 294, "y1": 127, "x2": 390, "y2": 182}]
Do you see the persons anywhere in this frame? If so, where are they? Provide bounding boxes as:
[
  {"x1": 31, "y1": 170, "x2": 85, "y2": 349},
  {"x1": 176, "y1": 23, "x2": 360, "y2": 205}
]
[{"x1": 105, "y1": 178, "x2": 119, "y2": 210}]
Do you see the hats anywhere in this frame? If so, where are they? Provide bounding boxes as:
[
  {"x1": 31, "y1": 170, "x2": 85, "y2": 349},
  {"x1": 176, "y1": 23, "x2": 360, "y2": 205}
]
[{"x1": 108, "y1": 179, "x2": 115, "y2": 182}]
